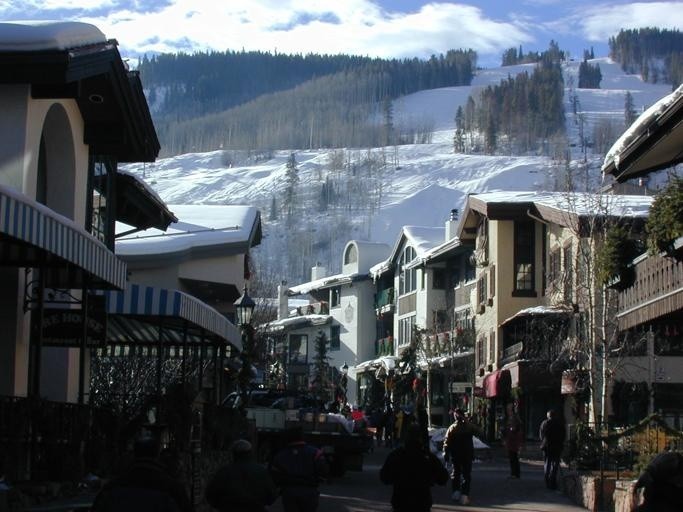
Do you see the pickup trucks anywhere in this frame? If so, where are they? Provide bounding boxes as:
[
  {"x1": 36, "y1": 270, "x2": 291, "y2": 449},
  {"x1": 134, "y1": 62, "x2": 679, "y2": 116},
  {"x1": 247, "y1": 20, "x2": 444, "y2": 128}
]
[{"x1": 220, "y1": 392, "x2": 347, "y2": 480}]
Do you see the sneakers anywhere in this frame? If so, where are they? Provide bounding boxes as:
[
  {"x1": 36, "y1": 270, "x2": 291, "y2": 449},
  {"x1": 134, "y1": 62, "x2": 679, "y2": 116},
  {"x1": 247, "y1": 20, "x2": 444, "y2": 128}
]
[{"x1": 451, "y1": 490, "x2": 470, "y2": 504}]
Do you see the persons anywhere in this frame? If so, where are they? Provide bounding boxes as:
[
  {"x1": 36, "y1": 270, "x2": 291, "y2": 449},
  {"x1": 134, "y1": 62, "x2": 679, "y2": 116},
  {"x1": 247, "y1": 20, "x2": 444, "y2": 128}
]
[
  {"x1": 499, "y1": 417, "x2": 523, "y2": 481},
  {"x1": 439, "y1": 407, "x2": 499, "y2": 508},
  {"x1": 624, "y1": 450, "x2": 682, "y2": 512},
  {"x1": 536, "y1": 408, "x2": 568, "y2": 491},
  {"x1": 84, "y1": 388, "x2": 452, "y2": 511}
]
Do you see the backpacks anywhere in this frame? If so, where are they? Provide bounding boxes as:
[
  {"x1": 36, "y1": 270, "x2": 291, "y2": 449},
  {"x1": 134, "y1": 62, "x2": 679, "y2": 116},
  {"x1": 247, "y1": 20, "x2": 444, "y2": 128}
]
[{"x1": 266, "y1": 440, "x2": 304, "y2": 475}]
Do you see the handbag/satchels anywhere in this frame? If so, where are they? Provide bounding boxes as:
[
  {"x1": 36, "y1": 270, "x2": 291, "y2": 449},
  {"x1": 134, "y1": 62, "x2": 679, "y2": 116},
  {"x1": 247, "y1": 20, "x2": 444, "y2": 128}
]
[{"x1": 380, "y1": 450, "x2": 403, "y2": 485}]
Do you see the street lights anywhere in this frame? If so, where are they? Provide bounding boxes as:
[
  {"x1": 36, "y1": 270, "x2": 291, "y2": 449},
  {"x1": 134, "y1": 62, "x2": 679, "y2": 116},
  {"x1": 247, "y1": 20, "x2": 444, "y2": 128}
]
[{"x1": 340, "y1": 361, "x2": 348, "y2": 406}]
